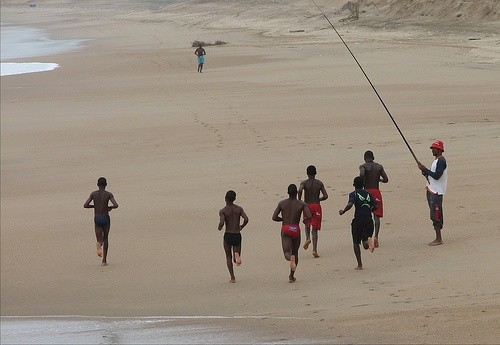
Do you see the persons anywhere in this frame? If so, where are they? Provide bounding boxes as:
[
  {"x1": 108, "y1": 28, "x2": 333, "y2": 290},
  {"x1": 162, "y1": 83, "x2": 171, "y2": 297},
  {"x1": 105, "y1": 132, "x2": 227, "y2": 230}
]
[
  {"x1": 298, "y1": 165, "x2": 328, "y2": 257},
  {"x1": 339, "y1": 177, "x2": 378, "y2": 270},
  {"x1": 272, "y1": 183, "x2": 313, "y2": 281},
  {"x1": 218, "y1": 190, "x2": 249, "y2": 283},
  {"x1": 419, "y1": 140, "x2": 447, "y2": 246},
  {"x1": 360, "y1": 151, "x2": 389, "y2": 247},
  {"x1": 195, "y1": 44, "x2": 206, "y2": 73},
  {"x1": 83, "y1": 177, "x2": 119, "y2": 263}
]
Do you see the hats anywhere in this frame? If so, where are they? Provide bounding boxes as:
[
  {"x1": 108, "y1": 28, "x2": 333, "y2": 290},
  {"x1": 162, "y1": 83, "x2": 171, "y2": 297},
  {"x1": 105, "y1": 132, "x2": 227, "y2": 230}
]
[{"x1": 429, "y1": 139, "x2": 445, "y2": 152}]
